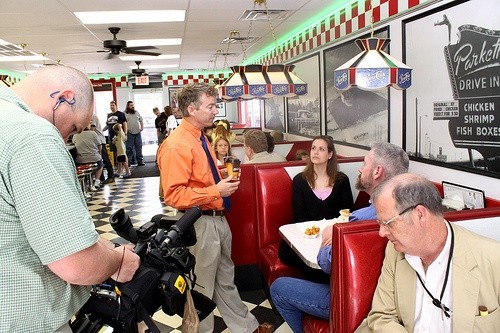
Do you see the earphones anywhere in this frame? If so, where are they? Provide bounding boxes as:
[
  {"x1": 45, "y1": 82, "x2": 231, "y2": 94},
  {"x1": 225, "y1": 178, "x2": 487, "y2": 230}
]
[{"x1": 53, "y1": 98, "x2": 65, "y2": 110}]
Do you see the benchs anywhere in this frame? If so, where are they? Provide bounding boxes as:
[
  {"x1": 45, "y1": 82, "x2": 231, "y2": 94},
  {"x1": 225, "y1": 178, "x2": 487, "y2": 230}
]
[{"x1": 227, "y1": 128, "x2": 500, "y2": 333}]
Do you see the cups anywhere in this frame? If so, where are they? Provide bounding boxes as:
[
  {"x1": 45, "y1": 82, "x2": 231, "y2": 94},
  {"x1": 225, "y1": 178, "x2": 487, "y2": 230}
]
[{"x1": 224, "y1": 159, "x2": 241, "y2": 181}]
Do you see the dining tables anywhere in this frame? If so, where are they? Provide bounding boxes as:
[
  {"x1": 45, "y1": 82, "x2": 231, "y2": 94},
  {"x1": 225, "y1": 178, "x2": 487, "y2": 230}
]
[{"x1": 279, "y1": 217, "x2": 351, "y2": 270}]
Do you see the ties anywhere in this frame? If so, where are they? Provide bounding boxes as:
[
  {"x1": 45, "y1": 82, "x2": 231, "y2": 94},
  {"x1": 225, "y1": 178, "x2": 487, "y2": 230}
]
[{"x1": 199, "y1": 131, "x2": 232, "y2": 213}]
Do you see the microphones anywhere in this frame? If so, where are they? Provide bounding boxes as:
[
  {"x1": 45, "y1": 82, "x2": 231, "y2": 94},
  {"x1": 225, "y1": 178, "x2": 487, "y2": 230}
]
[{"x1": 160, "y1": 207, "x2": 203, "y2": 250}]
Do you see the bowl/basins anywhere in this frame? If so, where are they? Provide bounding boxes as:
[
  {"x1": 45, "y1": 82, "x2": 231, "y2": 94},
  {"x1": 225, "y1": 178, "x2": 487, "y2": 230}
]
[{"x1": 304, "y1": 229, "x2": 318, "y2": 238}]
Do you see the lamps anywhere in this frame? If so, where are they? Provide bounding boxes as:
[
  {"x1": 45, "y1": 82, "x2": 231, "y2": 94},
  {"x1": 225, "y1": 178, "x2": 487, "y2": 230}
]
[
  {"x1": 201, "y1": 0, "x2": 308, "y2": 103},
  {"x1": 333, "y1": 0, "x2": 414, "y2": 91}
]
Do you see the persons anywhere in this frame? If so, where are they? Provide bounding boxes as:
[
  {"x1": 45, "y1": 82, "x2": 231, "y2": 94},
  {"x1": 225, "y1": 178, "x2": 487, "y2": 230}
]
[
  {"x1": 124, "y1": 101, "x2": 145, "y2": 166},
  {"x1": 64, "y1": 100, "x2": 138, "y2": 191},
  {"x1": 155, "y1": 81, "x2": 275, "y2": 333},
  {"x1": 270, "y1": 142, "x2": 410, "y2": 333},
  {"x1": 366, "y1": 173, "x2": 500, "y2": 333},
  {"x1": 204, "y1": 119, "x2": 310, "y2": 181},
  {"x1": 154, "y1": 106, "x2": 179, "y2": 146},
  {"x1": 0, "y1": 63, "x2": 140, "y2": 333},
  {"x1": 291, "y1": 136, "x2": 354, "y2": 285}
]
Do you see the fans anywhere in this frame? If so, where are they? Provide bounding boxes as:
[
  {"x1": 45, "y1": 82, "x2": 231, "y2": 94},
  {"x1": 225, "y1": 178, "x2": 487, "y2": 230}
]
[
  {"x1": 63, "y1": 27, "x2": 162, "y2": 60},
  {"x1": 109, "y1": 61, "x2": 166, "y2": 80}
]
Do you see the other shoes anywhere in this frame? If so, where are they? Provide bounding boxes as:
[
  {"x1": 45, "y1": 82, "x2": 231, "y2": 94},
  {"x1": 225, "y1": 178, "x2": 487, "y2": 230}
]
[
  {"x1": 139, "y1": 160, "x2": 146, "y2": 166},
  {"x1": 95, "y1": 179, "x2": 101, "y2": 187},
  {"x1": 103, "y1": 177, "x2": 114, "y2": 184},
  {"x1": 252, "y1": 321, "x2": 275, "y2": 333}
]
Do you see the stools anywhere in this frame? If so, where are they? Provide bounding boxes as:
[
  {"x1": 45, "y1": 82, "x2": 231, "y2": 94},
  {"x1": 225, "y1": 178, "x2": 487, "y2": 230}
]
[{"x1": 76, "y1": 163, "x2": 99, "y2": 199}]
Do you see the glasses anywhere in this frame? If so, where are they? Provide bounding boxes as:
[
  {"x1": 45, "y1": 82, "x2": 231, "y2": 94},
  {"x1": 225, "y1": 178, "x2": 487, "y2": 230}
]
[{"x1": 376, "y1": 203, "x2": 431, "y2": 227}]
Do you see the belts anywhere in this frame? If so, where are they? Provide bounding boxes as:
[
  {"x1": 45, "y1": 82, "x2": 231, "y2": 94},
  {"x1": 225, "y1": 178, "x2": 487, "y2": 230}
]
[{"x1": 179, "y1": 210, "x2": 226, "y2": 217}]
